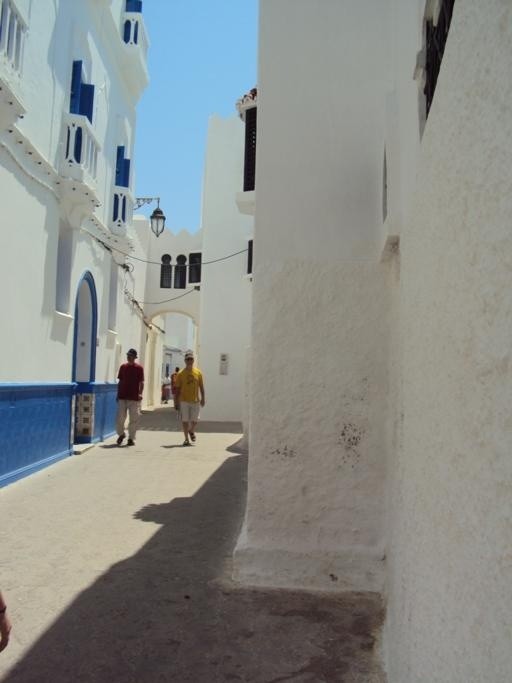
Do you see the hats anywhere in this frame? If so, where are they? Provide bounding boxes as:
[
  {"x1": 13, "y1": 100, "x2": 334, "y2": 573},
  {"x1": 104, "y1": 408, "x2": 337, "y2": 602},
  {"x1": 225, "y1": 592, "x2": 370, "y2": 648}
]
[
  {"x1": 126, "y1": 349, "x2": 138, "y2": 358},
  {"x1": 184, "y1": 352, "x2": 194, "y2": 360}
]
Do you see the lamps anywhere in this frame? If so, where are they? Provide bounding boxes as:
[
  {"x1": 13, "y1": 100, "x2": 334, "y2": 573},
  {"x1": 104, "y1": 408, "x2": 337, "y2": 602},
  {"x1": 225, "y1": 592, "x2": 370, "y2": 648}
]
[{"x1": 150, "y1": 198, "x2": 166, "y2": 237}]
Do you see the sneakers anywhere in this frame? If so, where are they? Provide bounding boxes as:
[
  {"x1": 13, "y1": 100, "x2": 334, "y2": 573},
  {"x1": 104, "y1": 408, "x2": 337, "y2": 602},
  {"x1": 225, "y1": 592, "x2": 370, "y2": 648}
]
[{"x1": 116, "y1": 432, "x2": 135, "y2": 445}]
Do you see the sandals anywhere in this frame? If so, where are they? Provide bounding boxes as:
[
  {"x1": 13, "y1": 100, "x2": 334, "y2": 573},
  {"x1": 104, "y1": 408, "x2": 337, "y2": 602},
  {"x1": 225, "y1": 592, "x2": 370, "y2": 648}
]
[{"x1": 183, "y1": 430, "x2": 197, "y2": 446}]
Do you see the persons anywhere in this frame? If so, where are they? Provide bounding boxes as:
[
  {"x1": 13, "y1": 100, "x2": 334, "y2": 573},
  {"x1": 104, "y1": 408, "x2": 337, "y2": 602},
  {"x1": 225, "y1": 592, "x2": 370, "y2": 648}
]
[
  {"x1": 0, "y1": 591, "x2": 13, "y2": 653},
  {"x1": 115, "y1": 348, "x2": 145, "y2": 446},
  {"x1": 173, "y1": 348, "x2": 206, "y2": 446},
  {"x1": 170, "y1": 367, "x2": 180, "y2": 410},
  {"x1": 161, "y1": 373, "x2": 172, "y2": 404}
]
